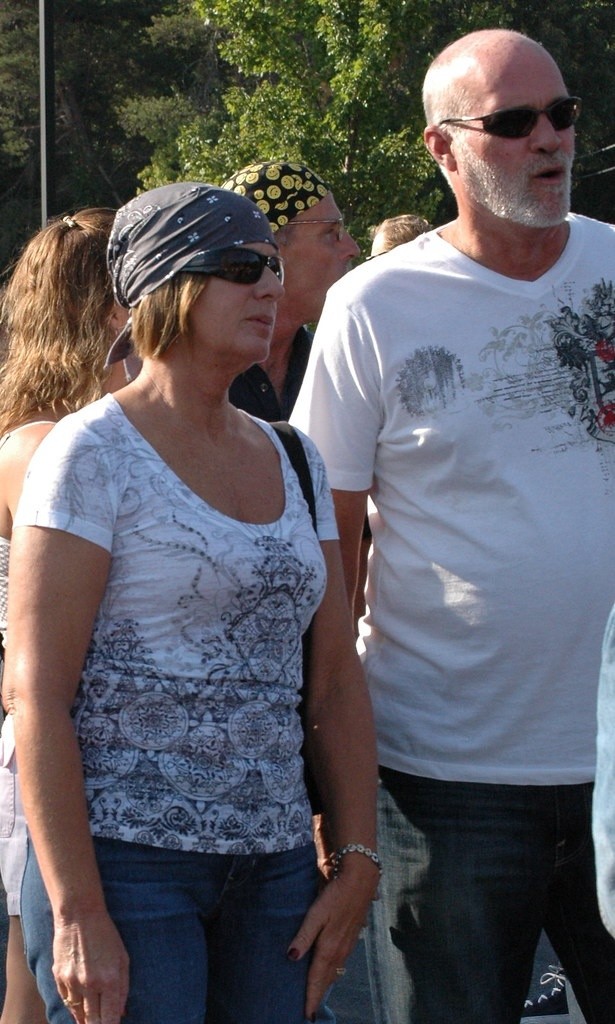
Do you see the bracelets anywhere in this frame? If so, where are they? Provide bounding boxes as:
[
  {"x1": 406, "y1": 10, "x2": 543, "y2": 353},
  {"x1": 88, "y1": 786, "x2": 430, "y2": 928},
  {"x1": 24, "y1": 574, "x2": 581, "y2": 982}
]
[{"x1": 333, "y1": 843, "x2": 383, "y2": 883}]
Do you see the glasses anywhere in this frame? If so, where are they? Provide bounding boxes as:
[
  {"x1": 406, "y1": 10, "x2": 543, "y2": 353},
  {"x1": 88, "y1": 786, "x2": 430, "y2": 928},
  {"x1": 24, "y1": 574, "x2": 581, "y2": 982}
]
[
  {"x1": 286, "y1": 218, "x2": 344, "y2": 242},
  {"x1": 179, "y1": 247, "x2": 285, "y2": 286},
  {"x1": 437, "y1": 97, "x2": 584, "y2": 138}
]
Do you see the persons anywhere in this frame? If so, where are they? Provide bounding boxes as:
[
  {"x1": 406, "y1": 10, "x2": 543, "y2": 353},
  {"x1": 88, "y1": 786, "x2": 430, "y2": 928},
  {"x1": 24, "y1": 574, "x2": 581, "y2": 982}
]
[{"x1": 0, "y1": 30, "x2": 614, "y2": 1024}]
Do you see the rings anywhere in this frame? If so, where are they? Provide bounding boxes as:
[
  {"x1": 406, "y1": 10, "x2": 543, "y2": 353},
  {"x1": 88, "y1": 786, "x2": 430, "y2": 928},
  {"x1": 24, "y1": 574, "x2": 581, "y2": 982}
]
[
  {"x1": 336, "y1": 967, "x2": 346, "y2": 975},
  {"x1": 62, "y1": 998, "x2": 81, "y2": 1007}
]
